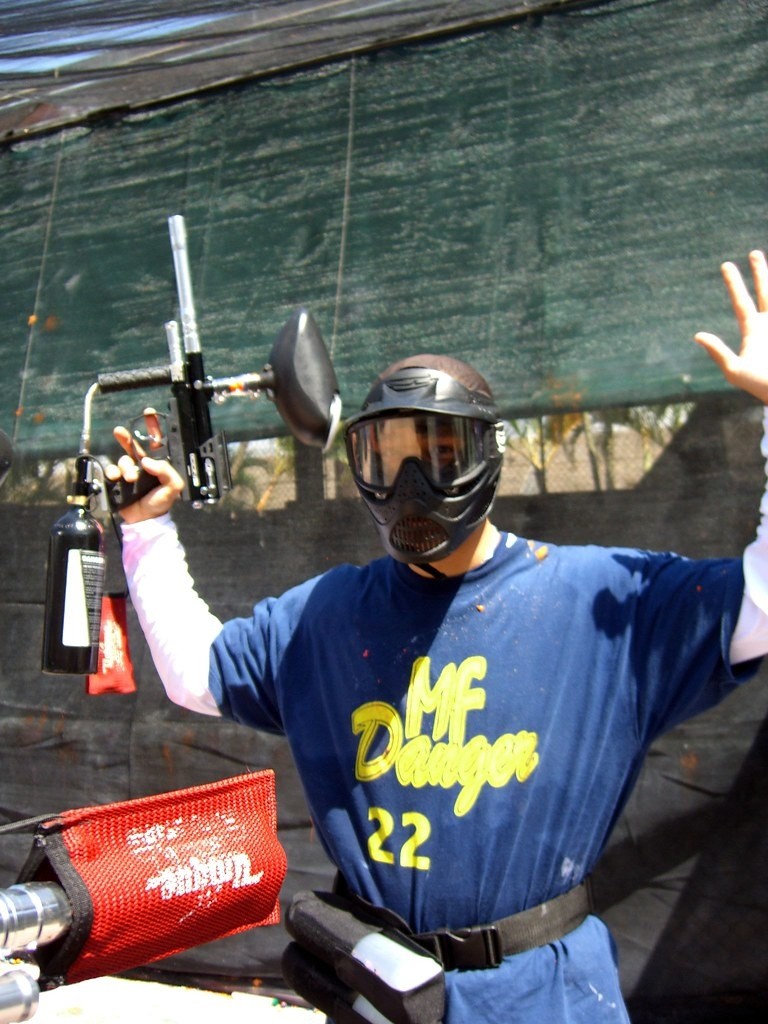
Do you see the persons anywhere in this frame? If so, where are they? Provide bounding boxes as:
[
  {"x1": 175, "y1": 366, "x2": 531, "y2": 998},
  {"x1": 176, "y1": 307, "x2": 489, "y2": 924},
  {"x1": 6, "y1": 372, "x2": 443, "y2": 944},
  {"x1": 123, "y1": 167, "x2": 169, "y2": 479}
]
[{"x1": 104, "y1": 246, "x2": 768, "y2": 1024}]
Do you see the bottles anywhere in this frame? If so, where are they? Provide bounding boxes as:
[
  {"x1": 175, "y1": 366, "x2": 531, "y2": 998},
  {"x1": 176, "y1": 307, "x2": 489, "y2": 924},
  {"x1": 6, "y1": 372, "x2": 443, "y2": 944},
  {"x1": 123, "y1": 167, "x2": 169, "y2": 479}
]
[{"x1": 40, "y1": 481, "x2": 105, "y2": 674}]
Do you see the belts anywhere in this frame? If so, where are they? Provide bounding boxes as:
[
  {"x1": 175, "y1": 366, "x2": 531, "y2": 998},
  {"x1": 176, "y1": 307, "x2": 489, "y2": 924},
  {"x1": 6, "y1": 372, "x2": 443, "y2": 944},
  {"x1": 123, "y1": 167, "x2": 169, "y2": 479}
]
[{"x1": 332, "y1": 881, "x2": 590, "y2": 969}]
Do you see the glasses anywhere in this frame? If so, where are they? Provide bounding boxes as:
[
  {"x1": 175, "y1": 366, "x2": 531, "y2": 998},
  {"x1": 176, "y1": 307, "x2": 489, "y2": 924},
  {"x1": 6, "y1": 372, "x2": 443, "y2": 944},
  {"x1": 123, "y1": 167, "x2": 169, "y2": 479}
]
[{"x1": 345, "y1": 407, "x2": 491, "y2": 491}]
